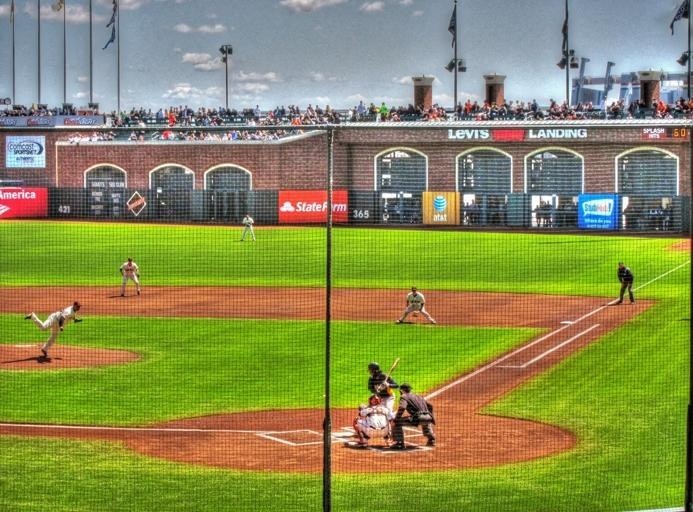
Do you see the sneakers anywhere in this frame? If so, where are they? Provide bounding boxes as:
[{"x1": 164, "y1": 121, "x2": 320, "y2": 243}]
[
  {"x1": 25, "y1": 312, "x2": 33, "y2": 320},
  {"x1": 384, "y1": 438, "x2": 406, "y2": 449},
  {"x1": 431, "y1": 321, "x2": 436, "y2": 324},
  {"x1": 41, "y1": 349, "x2": 48, "y2": 356},
  {"x1": 396, "y1": 320, "x2": 403, "y2": 324},
  {"x1": 120, "y1": 293, "x2": 125, "y2": 296},
  {"x1": 629, "y1": 301, "x2": 635, "y2": 305},
  {"x1": 137, "y1": 290, "x2": 140, "y2": 295},
  {"x1": 617, "y1": 301, "x2": 624, "y2": 305},
  {"x1": 425, "y1": 438, "x2": 436, "y2": 446},
  {"x1": 353, "y1": 440, "x2": 369, "y2": 447}
]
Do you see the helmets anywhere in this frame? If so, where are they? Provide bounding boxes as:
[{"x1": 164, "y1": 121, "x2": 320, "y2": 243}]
[
  {"x1": 367, "y1": 362, "x2": 379, "y2": 375},
  {"x1": 368, "y1": 394, "x2": 382, "y2": 406}
]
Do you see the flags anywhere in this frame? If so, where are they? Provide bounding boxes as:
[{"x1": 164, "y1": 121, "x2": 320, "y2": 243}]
[
  {"x1": 54, "y1": 0, "x2": 64, "y2": 12},
  {"x1": 447, "y1": 4, "x2": 456, "y2": 48},
  {"x1": 670, "y1": 0, "x2": 690, "y2": 35},
  {"x1": 9, "y1": 1, "x2": 16, "y2": 25},
  {"x1": 100, "y1": 1, "x2": 118, "y2": 49},
  {"x1": 561, "y1": 19, "x2": 567, "y2": 54}
]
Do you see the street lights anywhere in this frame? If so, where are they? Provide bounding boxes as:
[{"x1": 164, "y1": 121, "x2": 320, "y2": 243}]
[
  {"x1": 219, "y1": 44, "x2": 232, "y2": 110},
  {"x1": 677, "y1": 50, "x2": 691, "y2": 100},
  {"x1": 445, "y1": 57, "x2": 467, "y2": 113},
  {"x1": 556, "y1": 49, "x2": 581, "y2": 107}
]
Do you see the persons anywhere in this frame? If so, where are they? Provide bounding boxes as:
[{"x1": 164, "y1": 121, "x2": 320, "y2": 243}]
[
  {"x1": 462, "y1": 193, "x2": 674, "y2": 231},
  {"x1": 238, "y1": 214, "x2": 258, "y2": 243},
  {"x1": 392, "y1": 285, "x2": 438, "y2": 325},
  {"x1": 118, "y1": 257, "x2": 142, "y2": 297},
  {"x1": 389, "y1": 382, "x2": 436, "y2": 450},
  {"x1": 350, "y1": 394, "x2": 396, "y2": 448},
  {"x1": 347, "y1": 97, "x2": 693, "y2": 123},
  {"x1": 23, "y1": 300, "x2": 81, "y2": 361},
  {"x1": 365, "y1": 361, "x2": 399, "y2": 441},
  {"x1": 92, "y1": 103, "x2": 343, "y2": 143},
  {"x1": 4, "y1": 107, "x2": 78, "y2": 117},
  {"x1": 616, "y1": 261, "x2": 636, "y2": 307}
]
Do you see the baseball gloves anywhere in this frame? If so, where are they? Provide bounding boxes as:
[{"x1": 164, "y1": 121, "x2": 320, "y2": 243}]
[
  {"x1": 354, "y1": 416, "x2": 361, "y2": 426},
  {"x1": 413, "y1": 310, "x2": 420, "y2": 317}
]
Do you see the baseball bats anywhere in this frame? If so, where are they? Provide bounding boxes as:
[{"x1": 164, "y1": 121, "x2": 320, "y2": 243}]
[{"x1": 386, "y1": 358, "x2": 399, "y2": 381}]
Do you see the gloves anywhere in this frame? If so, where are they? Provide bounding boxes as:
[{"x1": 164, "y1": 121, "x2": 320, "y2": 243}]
[{"x1": 375, "y1": 380, "x2": 390, "y2": 392}]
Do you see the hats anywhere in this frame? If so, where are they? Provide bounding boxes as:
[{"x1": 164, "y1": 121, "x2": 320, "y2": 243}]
[
  {"x1": 412, "y1": 287, "x2": 417, "y2": 291},
  {"x1": 73, "y1": 301, "x2": 82, "y2": 307}
]
